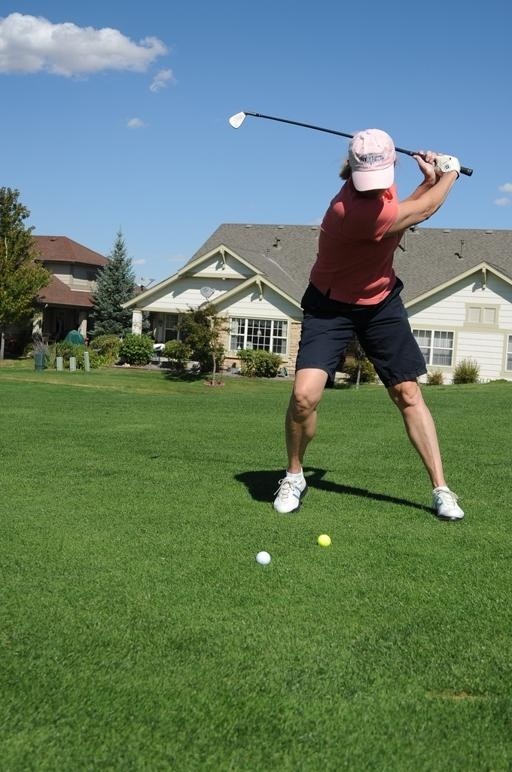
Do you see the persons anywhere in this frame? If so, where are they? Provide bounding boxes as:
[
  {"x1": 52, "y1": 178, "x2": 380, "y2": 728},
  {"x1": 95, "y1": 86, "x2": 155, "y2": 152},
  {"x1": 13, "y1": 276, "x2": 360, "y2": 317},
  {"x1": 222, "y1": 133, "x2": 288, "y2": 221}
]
[{"x1": 270, "y1": 127, "x2": 466, "y2": 523}]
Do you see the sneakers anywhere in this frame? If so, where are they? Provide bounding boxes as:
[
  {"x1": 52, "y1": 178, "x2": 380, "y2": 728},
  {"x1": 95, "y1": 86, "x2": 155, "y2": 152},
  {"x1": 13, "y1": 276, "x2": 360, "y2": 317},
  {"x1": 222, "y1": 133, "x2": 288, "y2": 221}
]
[
  {"x1": 273, "y1": 467, "x2": 309, "y2": 514},
  {"x1": 431, "y1": 485, "x2": 465, "y2": 521}
]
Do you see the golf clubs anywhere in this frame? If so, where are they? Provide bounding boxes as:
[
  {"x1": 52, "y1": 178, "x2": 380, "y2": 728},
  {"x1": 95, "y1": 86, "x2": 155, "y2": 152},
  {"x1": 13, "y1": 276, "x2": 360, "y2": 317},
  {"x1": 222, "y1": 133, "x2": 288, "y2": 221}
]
[{"x1": 228, "y1": 111, "x2": 473, "y2": 177}]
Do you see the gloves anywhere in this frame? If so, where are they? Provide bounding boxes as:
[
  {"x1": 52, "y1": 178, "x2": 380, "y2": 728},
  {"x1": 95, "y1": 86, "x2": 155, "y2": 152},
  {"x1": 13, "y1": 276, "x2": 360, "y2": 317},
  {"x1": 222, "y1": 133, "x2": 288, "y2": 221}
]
[{"x1": 435, "y1": 153, "x2": 462, "y2": 180}]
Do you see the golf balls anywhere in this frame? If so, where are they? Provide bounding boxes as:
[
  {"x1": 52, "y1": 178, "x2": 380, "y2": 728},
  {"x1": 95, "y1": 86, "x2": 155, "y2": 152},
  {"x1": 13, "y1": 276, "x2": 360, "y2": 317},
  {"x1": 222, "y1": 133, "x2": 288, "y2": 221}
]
[
  {"x1": 318, "y1": 535, "x2": 330, "y2": 546},
  {"x1": 256, "y1": 551, "x2": 271, "y2": 565}
]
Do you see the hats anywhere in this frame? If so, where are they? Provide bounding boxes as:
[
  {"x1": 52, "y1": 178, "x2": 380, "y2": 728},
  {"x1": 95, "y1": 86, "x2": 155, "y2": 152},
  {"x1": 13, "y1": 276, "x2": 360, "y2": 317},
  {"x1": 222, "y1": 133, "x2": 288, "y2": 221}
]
[{"x1": 347, "y1": 127, "x2": 397, "y2": 193}]
